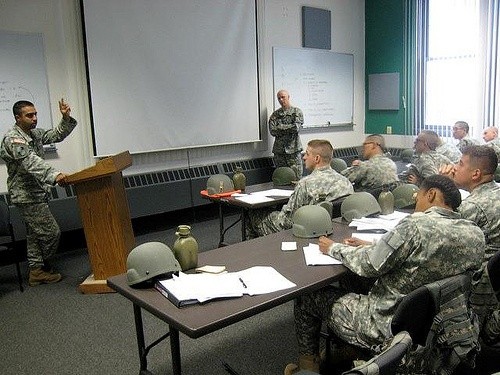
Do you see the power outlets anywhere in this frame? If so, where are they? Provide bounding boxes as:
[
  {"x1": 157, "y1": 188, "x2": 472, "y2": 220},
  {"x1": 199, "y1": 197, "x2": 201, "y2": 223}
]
[{"x1": 386, "y1": 125, "x2": 392, "y2": 134}]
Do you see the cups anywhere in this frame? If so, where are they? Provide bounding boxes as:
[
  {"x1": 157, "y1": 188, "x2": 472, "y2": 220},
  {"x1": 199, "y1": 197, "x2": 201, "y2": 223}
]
[{"x1": 386, "y1": 126, "x2": 392, "y2": 135}]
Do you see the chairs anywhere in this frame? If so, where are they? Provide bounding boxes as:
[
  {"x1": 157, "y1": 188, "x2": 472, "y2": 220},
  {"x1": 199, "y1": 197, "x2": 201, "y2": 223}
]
[
  {"x1": 0, "y1": 201, "x2": 23, "y2": 294},
  {"x1": 223, "y1": 151, "x2": 500, "y2": 375}
]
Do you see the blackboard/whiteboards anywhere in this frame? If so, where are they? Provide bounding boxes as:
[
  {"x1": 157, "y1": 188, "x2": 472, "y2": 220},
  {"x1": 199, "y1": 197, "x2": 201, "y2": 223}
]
[
  {"x1": 273, "y1": 46, "x2": 355, "y2": 127},
  {"x1": 0, "y1": 32, "x2": 58, "y2": 153}
]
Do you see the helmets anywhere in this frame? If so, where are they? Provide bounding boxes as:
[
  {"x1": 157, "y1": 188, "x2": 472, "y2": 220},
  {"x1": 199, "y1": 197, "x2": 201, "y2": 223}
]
[
  {"x1": 272, "y1": 166, "x2": 297, "y2": 186},
  {"x1": 330, "y1": 158, "x2": 348, "y2": 173},
  {"x1": 292, "y1": 205, "x2": 333, "y2": 238},
  {"x1": 206, "y1": 174, "x2": 234, "y2": 194},
  {"x1": 126, "y1": 242, "x2": 182, "y2": 286},
  {"x1": 340, "y1": 192, "x2": 382, "y2": 222},
  {"x1": 401, "y1": 148, "x2": 415, "y2": 163},
  {"x1": 392, "y1": 184, "x2": 420, "y2": 209}
]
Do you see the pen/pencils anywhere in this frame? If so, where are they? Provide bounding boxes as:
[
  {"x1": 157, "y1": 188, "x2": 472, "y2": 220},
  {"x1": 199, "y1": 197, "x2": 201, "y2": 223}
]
[{"x1": 239, "y1": 278, "x2": 247, "y2": 288}]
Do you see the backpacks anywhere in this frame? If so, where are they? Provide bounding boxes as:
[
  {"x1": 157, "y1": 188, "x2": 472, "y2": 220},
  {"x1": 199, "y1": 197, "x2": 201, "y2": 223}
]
[{"x1": 398, "y1": 260, "x2": 500, "y2": 375}]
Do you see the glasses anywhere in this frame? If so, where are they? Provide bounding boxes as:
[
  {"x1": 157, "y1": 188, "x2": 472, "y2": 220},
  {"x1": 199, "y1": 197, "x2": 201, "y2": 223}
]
[
  {"x1": 452, "y1": 127, "x2": 464, "y2": 131},
  {"x1": 363, "y1": 142, "x2": 382, "y2": 148},
  {"x1": 416, "y1": 138, "x2": 426, "y2": 143}
]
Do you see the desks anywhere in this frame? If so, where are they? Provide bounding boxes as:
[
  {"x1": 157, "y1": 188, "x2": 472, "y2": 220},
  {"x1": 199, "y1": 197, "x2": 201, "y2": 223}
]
[
  {"x1": 332, "y1": 201, "x2": 418, "y2": 234},
  {"x1": 106, "y1": 219, "x2": 382, "y2": 375},
  {"x1": 200, "y1": 174, "x2": 308, "y2": 250}
]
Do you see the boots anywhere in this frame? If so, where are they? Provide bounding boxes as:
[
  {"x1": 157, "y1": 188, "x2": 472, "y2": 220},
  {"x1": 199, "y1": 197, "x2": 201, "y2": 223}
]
[
  {"x1": 28, "y1": 267, "x2": 62, "y2": 286},
  {"x1": 320, "y1": 343, "x2": 354, "y2": 364},
  {"x1": 283, "y1": 354, "x2": 320, "y2": 375}
]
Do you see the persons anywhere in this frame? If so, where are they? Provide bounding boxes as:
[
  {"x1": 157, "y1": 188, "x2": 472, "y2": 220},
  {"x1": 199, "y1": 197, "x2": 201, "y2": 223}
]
[
  {"x1": 340, "y1": 134, "x2": 400, "y2": 200},
  {"x1": 439, "y1": 144, "x2": 500, "y2": 325},
  {"x1": 482, "y1": 127, "x2": 500, "y2": 164},
  {"x1": 0, "y1": 97, "x2": 78, "y2": 286},
  {"x1": 246, "y1": 139, "x2": 354, "y2": 240},
  {"x1": 407, "y1": 130, "x2": 463, "y2": 186},
  {"x1": 269, "y1": 89, "x2": 304, "y2": 179},
  {"x1": 452, "y1": 121, "x2": 480, "y2": 152},
  {"x1": 284, "y1": 175, "x2": 485, "y2": 375}
]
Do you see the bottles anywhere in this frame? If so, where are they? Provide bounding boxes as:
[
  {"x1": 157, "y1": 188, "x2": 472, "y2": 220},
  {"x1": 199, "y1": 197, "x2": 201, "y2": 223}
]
[
  {"x1": 290, "y1": 162, "x2": 303, "y2": 181},
  {"x1": 378, "y1": 183, "x2": 394, "y2": 215},
  {"x1": 233, "y1": 166, "x2": 246, "y2": 191},
  {"x1": 314, "y1": 193, "x2": 333, "y2": 224},
  {"x1": 385, "y1": 147, "x2": 392, "y2": 159},
  {"x1": 173, "y1": 225, "x2": 198, "y2": 270}
]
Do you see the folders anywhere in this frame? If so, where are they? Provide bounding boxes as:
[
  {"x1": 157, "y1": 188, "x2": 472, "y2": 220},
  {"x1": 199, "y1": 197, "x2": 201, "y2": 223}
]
[{"x1": 154, "y1": 277, "x2": 199, "y2": 306}]
